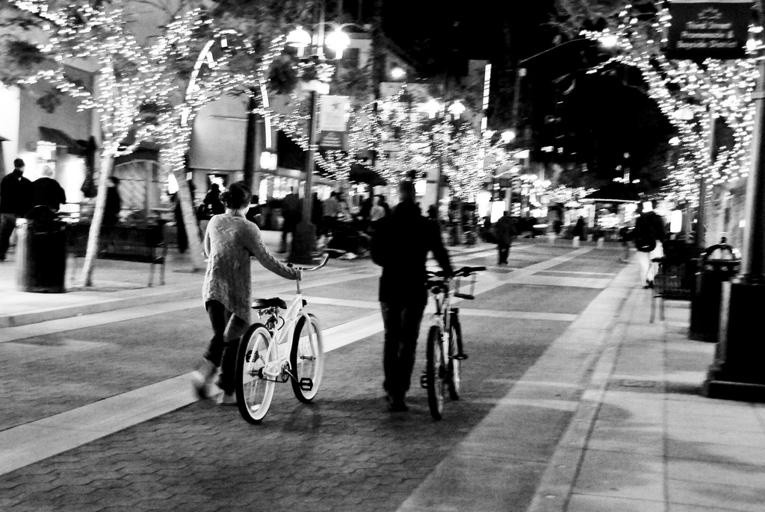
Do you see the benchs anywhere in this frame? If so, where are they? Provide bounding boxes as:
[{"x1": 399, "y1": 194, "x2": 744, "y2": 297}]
[
  {"x1": 70, "y1": 225, "x2": 166, "y2": 289},
  {"x1": 648, "y1": 259, "x2": 697, "y2": 328}
]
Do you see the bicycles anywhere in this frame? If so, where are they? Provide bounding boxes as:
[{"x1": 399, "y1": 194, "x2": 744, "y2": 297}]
[
  {"x1": 234, "y1": 254, "x2": 331, "y2": 425},
  {"x1": 421, "y1": 265, "x2": 488, "y2": 421}
]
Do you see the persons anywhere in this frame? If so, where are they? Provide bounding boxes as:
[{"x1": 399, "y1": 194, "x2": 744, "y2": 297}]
[
  {"x1": 192, "y1": 181, "x2": 306, "y2": 405},
  {"x1": 30, "y1": 164, "x2": 66, "y2": 220},
  {"x1": 170, "y1": 178, "x2": 225, "y2": 254},
  {"x1": 277, "y1": 186, "x2": 391, "y2": 257},
  {"x1": 635, "y1": 200, "x2": 667, "y2": 289},
  {"x1": 369, "y1": 180, "x2": 454, "y2": 406},
  {"x1": 100, "y1": 175, "x2": 124, "y2": 238},
  {"x1": 576, "y1": 215, "x2": 588, "y2": 241},
  {"x1": 492, "y1": 210, "x2": 516, "y2": 265},
  {"x1": 0, "y1": 158, "x2": 32, "y2": 261}
]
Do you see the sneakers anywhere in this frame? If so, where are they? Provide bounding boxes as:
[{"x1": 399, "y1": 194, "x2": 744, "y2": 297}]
[
  {"x1": 384, "y1": 391, "x2": 409, "y2": 412},
  {"x1": 216, "y1": 394, "x2": 237, "y2": 406}
]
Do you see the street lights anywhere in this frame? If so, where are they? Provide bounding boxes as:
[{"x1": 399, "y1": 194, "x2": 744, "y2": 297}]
[{"x1": 286, "y1": 26, "x2": 351, "y2": 264}]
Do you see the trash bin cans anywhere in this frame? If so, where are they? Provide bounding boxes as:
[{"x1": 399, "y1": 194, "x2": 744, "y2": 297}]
[
  {"x1": 15, "y1": 217, "x2": 69, "y2": 293},
  {"x1": 688, "y1": 243, "x2": 743, "y2": 343}
]
